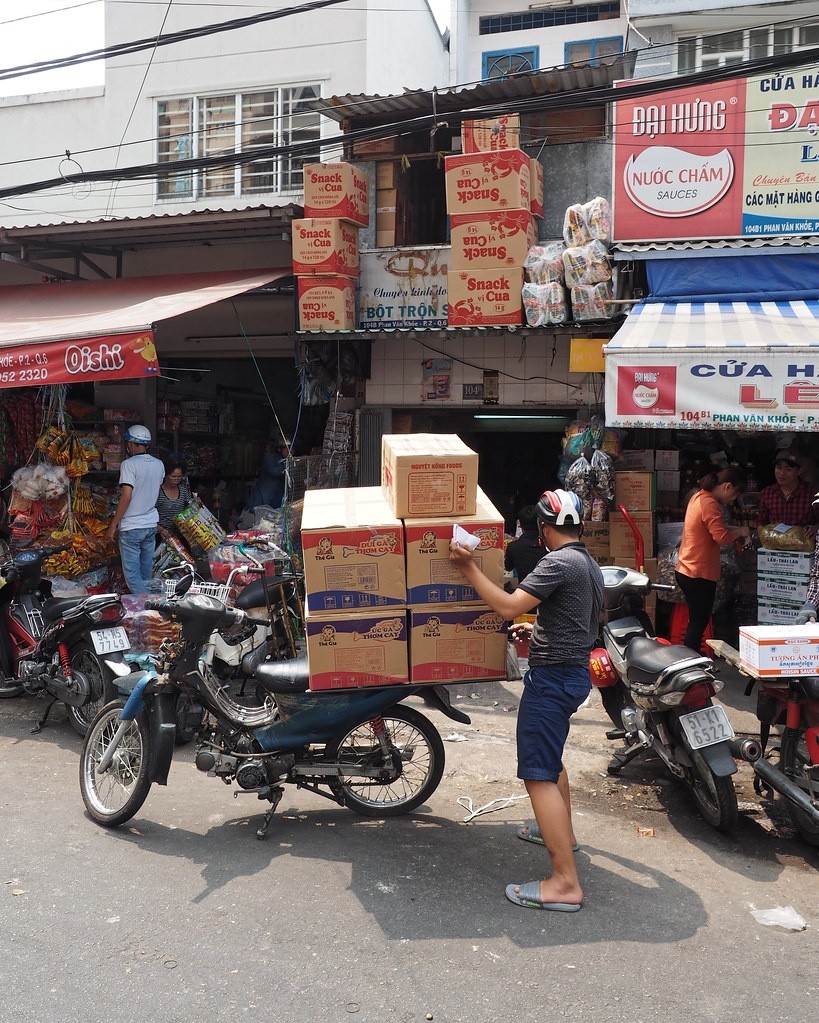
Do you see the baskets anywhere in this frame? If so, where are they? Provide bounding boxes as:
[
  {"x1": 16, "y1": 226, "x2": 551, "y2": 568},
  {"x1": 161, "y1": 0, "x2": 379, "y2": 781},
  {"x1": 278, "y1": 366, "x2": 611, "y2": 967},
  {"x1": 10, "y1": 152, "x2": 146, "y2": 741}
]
[{"x1": 165, "y1": 578, "x2": 232, "y2": 606}]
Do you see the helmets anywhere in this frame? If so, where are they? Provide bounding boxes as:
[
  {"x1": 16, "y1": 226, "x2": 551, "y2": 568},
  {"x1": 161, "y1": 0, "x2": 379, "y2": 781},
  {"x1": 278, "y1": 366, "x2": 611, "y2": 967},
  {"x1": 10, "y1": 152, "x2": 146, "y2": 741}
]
[
  {"x1": 535, "y1": 488, "x2": 583, "y2": 525},
  {"x1": 274, "y1": 433, "x2": 296, "y2": 449},
  {"x1": 124, "y1": 425, "x2": 151, "y2": 445},
  {"x1": 588, "y1": 648, "x2": 619, "y2": 688}
]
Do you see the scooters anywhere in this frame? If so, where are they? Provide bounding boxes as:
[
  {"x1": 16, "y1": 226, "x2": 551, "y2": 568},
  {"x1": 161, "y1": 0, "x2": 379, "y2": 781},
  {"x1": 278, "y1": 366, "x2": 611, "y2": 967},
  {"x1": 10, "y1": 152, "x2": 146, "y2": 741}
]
[
  {"x1": 745, "y1": 673, "x2": 819, "y2": 848},
  {"x1": 158, "y1": 561, "x2": 305, "y2": 744}
]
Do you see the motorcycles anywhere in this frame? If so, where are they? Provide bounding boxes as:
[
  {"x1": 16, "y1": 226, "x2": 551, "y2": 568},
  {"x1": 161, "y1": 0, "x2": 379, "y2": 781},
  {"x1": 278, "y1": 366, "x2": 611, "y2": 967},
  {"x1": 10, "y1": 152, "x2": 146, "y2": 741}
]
[
  {"x1": 0, "y1": 535, "x2": 132, "y2": 739},
  {"x1": 595, "y1": 565, "x2": 762, "y2": 835},
  {"x1": 79, "y1": 572, "x2": 472, "y2": 840}
]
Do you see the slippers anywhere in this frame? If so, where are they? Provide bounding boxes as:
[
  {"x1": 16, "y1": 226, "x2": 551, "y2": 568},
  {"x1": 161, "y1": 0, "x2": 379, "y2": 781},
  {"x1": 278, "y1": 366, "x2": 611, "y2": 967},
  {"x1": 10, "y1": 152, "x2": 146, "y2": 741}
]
[
  {"x1": 505, "y1": 880, "x2": 581, "y2": 912},
  {"x1": 517, "y1": 824, "x2": 579, "y2": 852}
]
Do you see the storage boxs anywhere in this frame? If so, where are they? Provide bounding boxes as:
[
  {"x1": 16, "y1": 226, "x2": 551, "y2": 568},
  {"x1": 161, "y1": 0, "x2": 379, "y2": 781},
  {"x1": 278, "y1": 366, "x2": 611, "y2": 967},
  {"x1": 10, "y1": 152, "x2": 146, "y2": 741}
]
[
  {"x1": 580, "y1": 450, "x2": 692, "y2": 631},
  {"x1": 738, "y1": 538, "x2": 819, "y2": 680},
  {"x1": 101, "y1": 401, "x2": 235, "y2": 471},
  {"x1": 297, "y1": 433, "x2": 506, "y2": 689},
  {"x1": 78, "y1": 565, "x2": 119, "y2": 618},
  {"x1": 291, "y1": 112, "x2": 544, "y2": 332}
]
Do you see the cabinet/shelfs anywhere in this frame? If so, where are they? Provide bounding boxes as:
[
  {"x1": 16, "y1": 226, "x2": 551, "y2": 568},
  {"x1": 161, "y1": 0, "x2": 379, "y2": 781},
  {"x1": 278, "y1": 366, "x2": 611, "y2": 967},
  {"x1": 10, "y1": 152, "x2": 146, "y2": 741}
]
[
  {"x1": 160, "y1": 432, "x2": 243, "y2": 479},
  {"x1": 45, "y1": 420, "x2": 143, "y2": 478}
]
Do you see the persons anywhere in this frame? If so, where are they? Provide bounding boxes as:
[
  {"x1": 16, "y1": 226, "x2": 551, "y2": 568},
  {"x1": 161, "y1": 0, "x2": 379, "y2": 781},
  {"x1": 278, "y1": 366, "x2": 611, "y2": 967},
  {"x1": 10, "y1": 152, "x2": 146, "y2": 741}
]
[
  {"x1": 105, "y1": 425, "x2": 165, "y2": 592},
  {"x1": 155, "y1": 459, "x2": 203, "y2": 540},
  {"x1": 674, "y1": 466, "x2": 751, "y2": 660},
  {"x1": 450, "y1": 488, "x2": 604, "y2": 912},
  {"x1": 251, "y1": 435, "x2": 295, "y2": 505},
  {"x1": 505, "y1": 506, "x2": 549, "y2": 583},
  {"x1": 758, "y1": 450, "x2": 819, "y2": 540}
]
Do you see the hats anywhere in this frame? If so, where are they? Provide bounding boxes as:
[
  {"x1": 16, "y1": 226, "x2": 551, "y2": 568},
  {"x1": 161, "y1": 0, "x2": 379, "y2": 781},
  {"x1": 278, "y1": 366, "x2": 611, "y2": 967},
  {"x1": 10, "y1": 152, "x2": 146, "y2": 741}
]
[{"x1": 776, "y1": 446, "x2": 801, "y2": 466}]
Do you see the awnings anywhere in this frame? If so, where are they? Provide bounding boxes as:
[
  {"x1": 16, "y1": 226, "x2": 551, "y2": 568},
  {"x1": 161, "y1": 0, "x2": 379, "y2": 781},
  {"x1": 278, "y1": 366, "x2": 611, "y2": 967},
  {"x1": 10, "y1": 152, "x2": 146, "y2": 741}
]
[
  {"x1": 0, "y1": 267, "x2": 295, "y2": 388},
  {"x1": 602, "y1": 298, "x2": 819, "y2": 431}
]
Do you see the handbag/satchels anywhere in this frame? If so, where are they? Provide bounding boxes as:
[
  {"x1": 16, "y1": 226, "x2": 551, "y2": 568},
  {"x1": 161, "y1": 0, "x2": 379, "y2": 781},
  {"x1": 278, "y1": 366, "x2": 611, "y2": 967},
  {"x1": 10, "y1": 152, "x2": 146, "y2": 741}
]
[
  {"x1": 760, "y1": 522, "x2": 815, "y2": 551},
  {"x1": 506, "y1": 642, "x2": 522, "y2": 681},
  {"x1": 562, "y1": 420, "x2": 620, "y2": 502}
]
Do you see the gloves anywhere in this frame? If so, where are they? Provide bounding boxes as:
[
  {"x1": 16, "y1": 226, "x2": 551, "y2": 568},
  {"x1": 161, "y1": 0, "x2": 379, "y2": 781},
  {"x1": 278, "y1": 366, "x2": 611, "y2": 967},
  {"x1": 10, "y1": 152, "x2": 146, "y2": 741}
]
[{"x1": 795, "y1": 603, "x2": 817, "y2": 625}]
